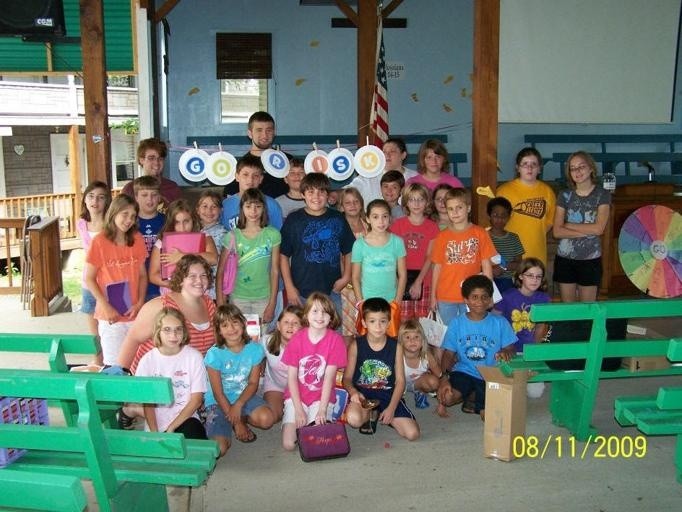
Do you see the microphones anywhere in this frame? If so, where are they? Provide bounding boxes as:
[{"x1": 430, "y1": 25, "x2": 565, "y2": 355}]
[{"x1": 642, "y1": 160, "x2": 654, "y2": 171}]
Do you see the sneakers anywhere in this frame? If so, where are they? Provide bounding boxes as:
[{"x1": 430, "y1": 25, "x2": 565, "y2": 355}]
[{"x1": 414, "y1": 391, "x2": 429, "y2": 409}]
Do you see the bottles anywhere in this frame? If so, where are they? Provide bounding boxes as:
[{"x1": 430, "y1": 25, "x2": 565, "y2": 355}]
[{"x1": 602, "y1": 172, "x2": 616, "y2": 195}]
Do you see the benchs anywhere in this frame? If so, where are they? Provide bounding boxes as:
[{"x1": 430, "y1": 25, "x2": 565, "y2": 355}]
[
  {"x1": 615, "y1": 338, "x2": 682, "y2": 483},
  {"x1": 0, "y1": 333, "x2": 122, "y2": 429},
  {"x1": 499, "y1": 299, "x2": 682, "y2": 443},
  {"x1": 0, "y1": 368, "x2": 219, "y2": 512}
]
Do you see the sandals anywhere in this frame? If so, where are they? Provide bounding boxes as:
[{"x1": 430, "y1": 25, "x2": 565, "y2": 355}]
[{"x1": 114, "y1": 406, "x2": 138, "y2": 432}]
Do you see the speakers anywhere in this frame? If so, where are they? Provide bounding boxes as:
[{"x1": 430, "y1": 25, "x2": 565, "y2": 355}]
[{"x1": 0, "y1": 0, "x2": 66, "y2": 41}]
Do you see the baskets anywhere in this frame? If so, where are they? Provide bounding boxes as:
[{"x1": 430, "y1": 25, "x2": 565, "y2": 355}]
[{"x1": 0, "y1": 397, "x2": 50, "y2": 469}]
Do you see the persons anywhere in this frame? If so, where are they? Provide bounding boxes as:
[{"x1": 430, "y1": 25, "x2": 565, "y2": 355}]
[
  {"x1": 438, "y1": 275, "x2": 519, "y2": 422},
  {"x1": 491, "y1": 255, "x2": 553, "y2": 399},
  {"x1": 428, "y1": 186, "x2": 498, "y2": 327},
  {"x1": 223, "y1": 110, "x2": 294, "y2": 199},
  {"x1": 486, "y1": 196, "x2": 525, "y2": 295},
  {"x1": 74, "y1": 138, "x2": 464, "y2": 456},
  {"x1": 497, "y1": 146, "x2": 557, "y2": 290},
  {"x1": 552, "y1": 149, "x2": 612, "y2": 302}
]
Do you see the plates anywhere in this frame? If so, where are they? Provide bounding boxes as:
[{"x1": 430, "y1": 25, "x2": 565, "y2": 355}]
[{"x1": 177, "y1": 145, "x2": 387, "y2": 186}]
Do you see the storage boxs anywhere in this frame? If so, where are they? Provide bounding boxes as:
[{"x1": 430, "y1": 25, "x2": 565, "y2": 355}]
[{"x1": 475, "y1": 365, "x2": 538, "y2": 463}]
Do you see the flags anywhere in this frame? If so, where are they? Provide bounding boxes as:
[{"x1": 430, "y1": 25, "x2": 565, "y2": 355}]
[{"x1": 367, "y1": 8, "x2": 389, "y2": 150}]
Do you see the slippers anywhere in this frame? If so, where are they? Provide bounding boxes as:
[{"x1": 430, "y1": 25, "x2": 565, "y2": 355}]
[
  {"x1": 462, "y1": 399, "x2": 476, "y2": 414},
  {"x1": 232, "y1": 423, "x2": 257, "y2": 443},
  {"x1": 358, "y1": 410, "x2": 380, "y2": 435}
]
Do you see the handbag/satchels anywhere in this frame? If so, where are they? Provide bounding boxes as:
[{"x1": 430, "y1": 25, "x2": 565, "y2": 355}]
[{"x1": 297, "y1": 418, "x2": 351, "y2": 463}]
